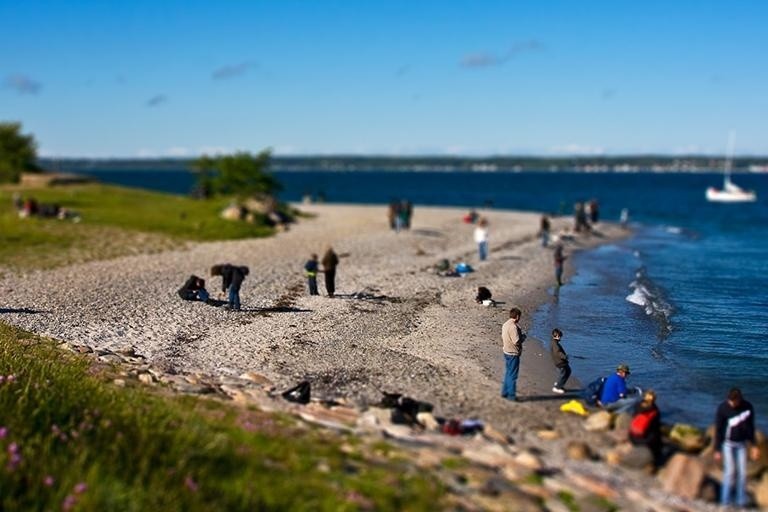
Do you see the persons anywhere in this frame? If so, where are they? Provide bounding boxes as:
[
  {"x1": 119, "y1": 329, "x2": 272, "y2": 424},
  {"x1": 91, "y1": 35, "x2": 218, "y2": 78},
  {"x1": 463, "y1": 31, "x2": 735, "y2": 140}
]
[
  {"x1": 539, "y1": 200, "x2": 601, "y2": 286},
  {"x1": 600, "y1": 363, "x2": 636, "y2": 419},
  {"x1": 386, "y1": 196, "x2": 412, "y2": 233},
  {"x1": 304, "y1": 253, "x2": 319, "y2": 295},
  {"x1": 501, "y1": 308, "x2": 527, "y2": 402},
  {"x1": 322, "y1": 247, "x2": 338, "y2": 296},
  {"x1": 179, "y1": 275, "x2": 215, "y2": 307},
  {"x1": 12, "y1": 195, "x2": 69, "y2": 220},
  {"x1": 714, "y1": 387, "x2": 760, "y2": 508},
  {"x1": 210, "y1": 263, "x2": 245, "y2": 312},
  {"x1": 628, "y1": 389, "x2": 666, "y2": 472},
  {"x1": 551, "y1": 328, "x2": 572, "y2": 394},
  {"x1": 473, "y1": 217, "x2": 490, "y2": 260}
]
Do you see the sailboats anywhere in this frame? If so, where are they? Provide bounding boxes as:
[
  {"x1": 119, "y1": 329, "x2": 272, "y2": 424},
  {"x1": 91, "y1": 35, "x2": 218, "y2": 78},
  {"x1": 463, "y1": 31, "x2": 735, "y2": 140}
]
[{"x1": 705, "y1": 130, "x2": 757, "y2": 203}]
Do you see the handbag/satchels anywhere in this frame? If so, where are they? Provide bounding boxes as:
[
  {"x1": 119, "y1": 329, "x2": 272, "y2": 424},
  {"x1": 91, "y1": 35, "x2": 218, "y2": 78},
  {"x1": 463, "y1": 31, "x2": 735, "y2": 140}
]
[{"x1": 630, "y1": 414, "x2": 650, "y2": 438}]
